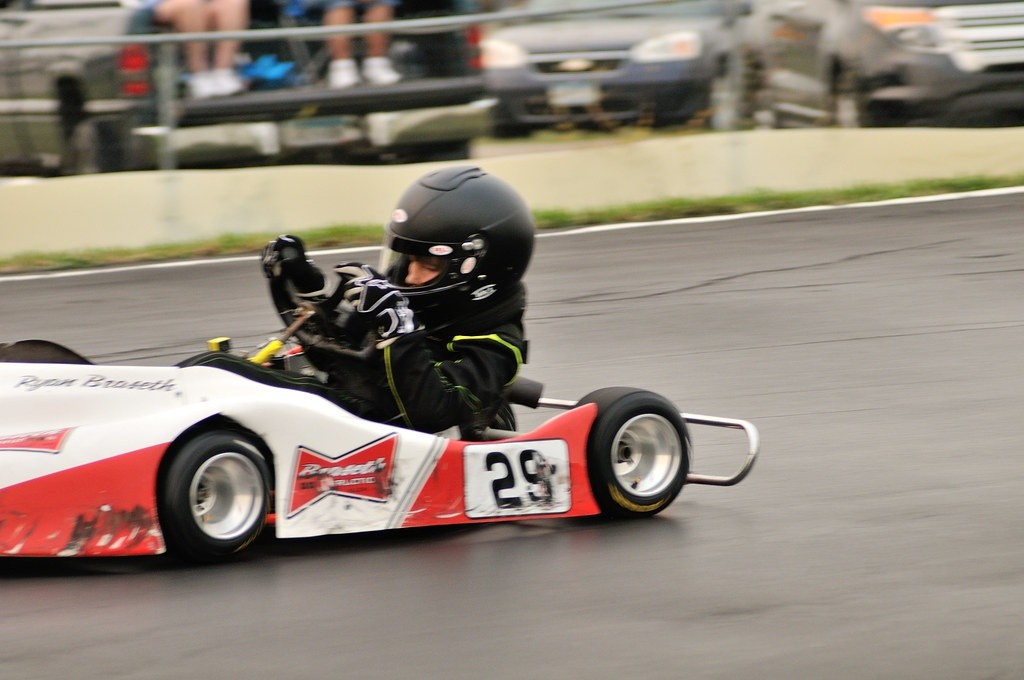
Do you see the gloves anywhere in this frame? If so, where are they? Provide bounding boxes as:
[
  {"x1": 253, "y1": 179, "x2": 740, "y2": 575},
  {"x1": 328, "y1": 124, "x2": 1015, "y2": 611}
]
[
  {"x1": 332, "y1": 259, "x2": 426, "y2": 340},
  {"x1": 258, "y1": 231, "x2": 330, "y2": 301}
]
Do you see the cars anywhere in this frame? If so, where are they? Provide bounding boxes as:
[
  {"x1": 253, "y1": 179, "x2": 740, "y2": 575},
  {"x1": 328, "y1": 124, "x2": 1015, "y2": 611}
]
[{"x1": 0, "y1": 0, "x2": 496, "y2": 175}]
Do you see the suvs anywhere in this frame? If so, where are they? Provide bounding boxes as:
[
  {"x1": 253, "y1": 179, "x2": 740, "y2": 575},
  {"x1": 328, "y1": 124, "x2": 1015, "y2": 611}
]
[
  {"x1": 478, "y1": 0, "x2": 754, "y2": 134},
  {"x1": 767, "y1": 1, "x2": 1024, "y2": 131}
]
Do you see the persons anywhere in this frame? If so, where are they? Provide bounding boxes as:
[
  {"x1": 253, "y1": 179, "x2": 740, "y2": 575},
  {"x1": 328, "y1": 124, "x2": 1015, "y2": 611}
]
[
  {"x1": 318, "y1": 0, "x2": 404, "y2": 89},
  {"x1": 125, "y1": 0, "x2": 250, "y2": 102},
  {"x1": 256, "y1": 166, "x2": 530, "y2": 443}
]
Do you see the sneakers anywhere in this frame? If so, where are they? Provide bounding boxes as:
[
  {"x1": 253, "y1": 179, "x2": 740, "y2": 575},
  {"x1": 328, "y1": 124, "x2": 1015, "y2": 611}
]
[
  {"x1": 185, "y1": 67, "x2": 246, "y2": 102},
  {"x1": 325, "y1": 57, "x2": 363, "y2": 91},
  {"x1": 361, "y1": 56, "x2": 403, "y2": 87}
]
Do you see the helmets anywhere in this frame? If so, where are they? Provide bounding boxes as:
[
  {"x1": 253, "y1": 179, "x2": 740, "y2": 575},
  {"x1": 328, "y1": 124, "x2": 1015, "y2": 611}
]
[{"x1": 381, "y1": 167, "x2": 537, "y2": 345}]
